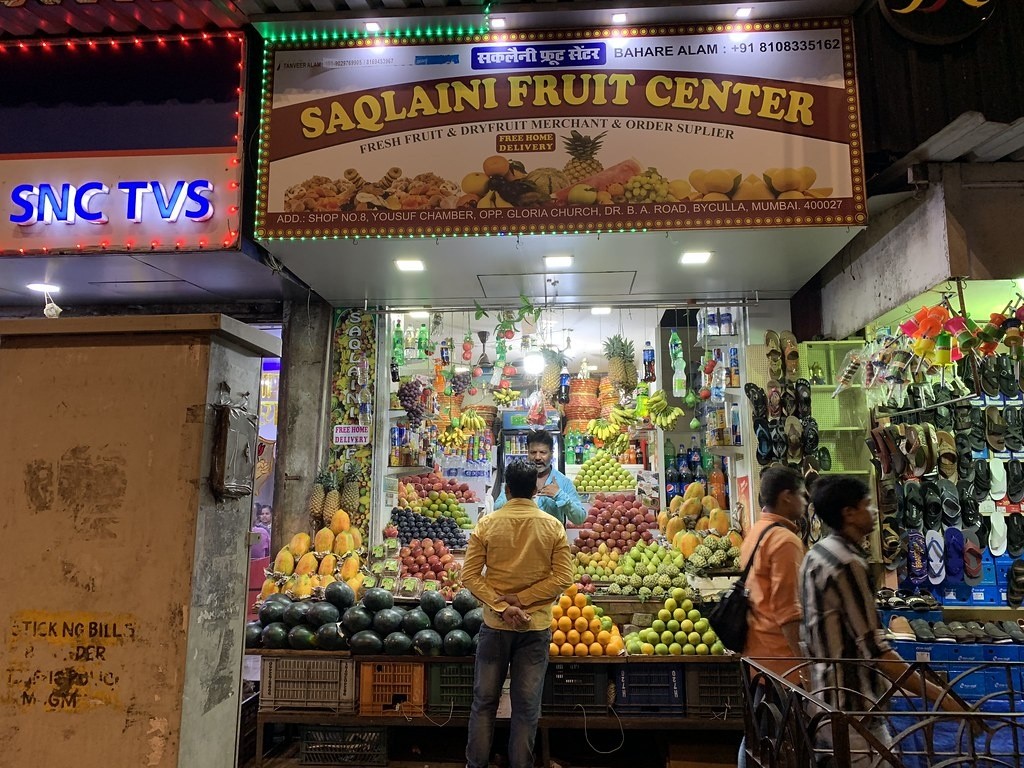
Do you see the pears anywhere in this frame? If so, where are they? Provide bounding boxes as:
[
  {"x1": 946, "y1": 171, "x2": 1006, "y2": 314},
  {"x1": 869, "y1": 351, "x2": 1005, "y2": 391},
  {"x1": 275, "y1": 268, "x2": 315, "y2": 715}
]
[
  {"x1": 398, "y1": 481, "x2": 421, "y2": 515},
  {"x1": 444, "y1": 386, "x2": 460, "y2": 427},
  {"x1": 570, "y1": 537, "x2": 684, "y2": 581},
  {"x1": 685, "y1": 392, "x2": 701, "y2": 429}
]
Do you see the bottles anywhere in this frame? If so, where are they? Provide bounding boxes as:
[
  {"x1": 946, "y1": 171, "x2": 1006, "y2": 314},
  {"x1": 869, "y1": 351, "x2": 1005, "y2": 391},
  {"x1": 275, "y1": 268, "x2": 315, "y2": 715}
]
[
  {"x1": 672, "y1": 351, "x2": 687, "y2": 397},
  {"x1": 558, "y1": 364, "x2": 570, "y2": 403},
  {"x1": 643, "y1": 341, "x2": 656, "y2": 382},
  {"x1": 358, "y1": 384, "x2": 372, "y2": 426},
  {"x1": 434, "y1": 429, "x2": 495, "y2": 500},
  {"x1": 440, "y1": 341, "x2": 450, "y2": 366},
  {"x1": 668, "y1": 328, "x2": 683, "y2": 371},
  {"x1": 357, "y1": 352, "x2": 369, "y2": 386},
  {"x1": 390, "y1": 323, "x2": 429, "y2": 381},
  {"x1": 696, "y1": 307, "x2": 734, "y2": 341},
  {"x1": 400, "y1": 433, "x2": 434, "y2": 468},
  {"x1": 506, "y1": 455, "x2": 527, "y2": 464},
  {"x1": 710, "y1": 358, "x2": 726, "y2": 403},
  {"x1": 636, "y1": 379, "x2": 649, "y2": 417},
  {"x1": 730, "y1": 403, "x2": 740, "y2": 427},
  {"x1": 490, "y1": 356, "x2": 505, "y2": 386},
  {"x1": 564, "y1": 429, "x2": 644, "y2": 465},
  {"x1": 664, "y1": 435, "x2": 730, "y2": 510}
]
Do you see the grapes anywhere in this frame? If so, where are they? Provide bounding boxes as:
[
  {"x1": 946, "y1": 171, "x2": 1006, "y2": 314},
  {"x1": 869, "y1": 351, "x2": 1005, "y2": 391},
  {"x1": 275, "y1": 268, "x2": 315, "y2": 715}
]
[{"x1": 396, "y1": 372, "x2": 473, "y2": 433}]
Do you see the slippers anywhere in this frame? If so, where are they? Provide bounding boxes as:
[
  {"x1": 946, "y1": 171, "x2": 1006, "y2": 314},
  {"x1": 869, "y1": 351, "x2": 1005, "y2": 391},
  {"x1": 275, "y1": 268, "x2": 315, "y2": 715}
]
[
  {"x1": 865, "y1": 342, "x2": 1024, "y2": 613},
  {"x1": 743, "y1": 330, "x2": 832, "y2": 550}
]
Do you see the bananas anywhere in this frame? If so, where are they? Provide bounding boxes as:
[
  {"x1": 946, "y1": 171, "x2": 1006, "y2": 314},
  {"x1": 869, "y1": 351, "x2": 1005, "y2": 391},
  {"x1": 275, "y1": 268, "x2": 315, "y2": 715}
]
[
  {"x1": 492, "y1": 388, "x2": 521, "y2": 403},
  {"x1": 587, "y1": 389, "x2": 686, "y2": 457},
  {"x1": 437, "y1": 409, "x2": 486, "y2": 446}
]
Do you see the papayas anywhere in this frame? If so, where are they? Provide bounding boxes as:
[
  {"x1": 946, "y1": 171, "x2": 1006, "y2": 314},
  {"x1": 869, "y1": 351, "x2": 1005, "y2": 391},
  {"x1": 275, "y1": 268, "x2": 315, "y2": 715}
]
[
  {"x1": 658, "y1": 482, "x2": 744, "y2": 558},
  {"x1": 261, "y1": 508, "x2": 366, "y2": 600}
]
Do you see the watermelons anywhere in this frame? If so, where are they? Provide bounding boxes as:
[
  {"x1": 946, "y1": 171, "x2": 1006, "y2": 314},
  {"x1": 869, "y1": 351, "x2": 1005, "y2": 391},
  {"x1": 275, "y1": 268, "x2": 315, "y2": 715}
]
[{"x1": 245, "y1": 580, "x2": 482, "y2": 657}]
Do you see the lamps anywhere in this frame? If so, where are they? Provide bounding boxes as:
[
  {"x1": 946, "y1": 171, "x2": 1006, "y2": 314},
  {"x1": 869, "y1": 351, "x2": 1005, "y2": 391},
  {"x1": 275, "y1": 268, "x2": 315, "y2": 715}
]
[{"x1": 477, "y1": 331, "x2": 493, "y2": 368}]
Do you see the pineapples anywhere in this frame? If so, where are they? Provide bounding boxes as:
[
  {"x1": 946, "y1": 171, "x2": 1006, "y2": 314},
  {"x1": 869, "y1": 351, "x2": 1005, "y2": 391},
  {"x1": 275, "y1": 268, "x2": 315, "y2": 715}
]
[
  {"x1": 540, "y1": 349, "x2": 562, "y2": 394},
  {"x1": 602, "y1": 335, "x2": 638, "y2": 390},
  {"x1": 309, "y1": 463, "x2": 360, "y2": 525}
]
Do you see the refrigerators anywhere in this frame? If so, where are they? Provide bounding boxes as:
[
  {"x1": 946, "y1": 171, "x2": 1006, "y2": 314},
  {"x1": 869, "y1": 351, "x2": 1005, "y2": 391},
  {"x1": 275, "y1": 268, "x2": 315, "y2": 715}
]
[{"x1": 500, "y1": 411, "x2": 565, "y2": 484}]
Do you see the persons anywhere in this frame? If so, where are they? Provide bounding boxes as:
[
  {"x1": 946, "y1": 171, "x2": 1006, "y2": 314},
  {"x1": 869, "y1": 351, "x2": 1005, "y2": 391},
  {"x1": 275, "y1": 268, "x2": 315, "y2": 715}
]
[
  {"x1": 493, "y1": 430, "x2": 587, "y2": 533},
  {"x1": 250, "y1": 503, "x2": 273, "y2": 561},
  {"x1": 459, "y1": 460, "x2": 575, "y2": 768},
  {"x1": 737, "y1": 466, "x2": 973, "y2": 768}
]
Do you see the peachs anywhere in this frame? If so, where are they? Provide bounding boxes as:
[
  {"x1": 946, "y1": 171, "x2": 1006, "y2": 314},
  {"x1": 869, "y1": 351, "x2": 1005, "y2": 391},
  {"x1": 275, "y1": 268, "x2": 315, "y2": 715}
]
[{"x1": 395, "y1": 537, "x2": 462, "y2": 582}]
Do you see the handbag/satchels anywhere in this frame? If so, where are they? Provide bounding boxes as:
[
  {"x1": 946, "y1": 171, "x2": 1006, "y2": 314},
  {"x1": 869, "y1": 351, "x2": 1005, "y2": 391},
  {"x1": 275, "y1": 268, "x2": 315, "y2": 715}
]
[{"x1": 708, "y1": 582, "x2": 749, "y2": 655}]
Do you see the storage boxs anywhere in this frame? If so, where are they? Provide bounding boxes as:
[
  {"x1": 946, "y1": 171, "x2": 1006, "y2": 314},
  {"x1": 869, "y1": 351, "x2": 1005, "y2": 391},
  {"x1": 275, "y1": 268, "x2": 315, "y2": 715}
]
[
  {"x1": 873, "y1": 541, "x2": 1024, "y2": 700},
  {"x1": 242, "y1": 651, "x2": 747, "y2": 767}
]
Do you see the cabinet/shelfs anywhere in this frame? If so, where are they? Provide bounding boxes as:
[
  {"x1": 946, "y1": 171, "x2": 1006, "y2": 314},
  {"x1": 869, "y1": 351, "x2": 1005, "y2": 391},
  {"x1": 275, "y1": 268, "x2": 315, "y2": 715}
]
[{"x1": 793, "y1": 339, "x2": 870, "y2": 479}]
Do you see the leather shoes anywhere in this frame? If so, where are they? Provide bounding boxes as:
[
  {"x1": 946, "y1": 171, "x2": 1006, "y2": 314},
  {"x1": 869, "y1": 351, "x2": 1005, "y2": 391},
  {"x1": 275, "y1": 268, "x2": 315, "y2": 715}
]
[{"x1": 886, "y1": 614, "x2": 1024, "y2": 645}]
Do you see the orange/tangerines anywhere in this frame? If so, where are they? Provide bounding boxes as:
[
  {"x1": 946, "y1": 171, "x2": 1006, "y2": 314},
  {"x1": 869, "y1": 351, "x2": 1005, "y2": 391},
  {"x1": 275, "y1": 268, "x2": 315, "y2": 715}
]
[
  {"x1": 549, "y1": 583, "x2": 624, "y2": 657},
  {"x1": 624, "y1": 588, "x2": 724, "y2": 655},
  {"x1": 420, "y1": 491, "x2": 475, "y2": 528}
]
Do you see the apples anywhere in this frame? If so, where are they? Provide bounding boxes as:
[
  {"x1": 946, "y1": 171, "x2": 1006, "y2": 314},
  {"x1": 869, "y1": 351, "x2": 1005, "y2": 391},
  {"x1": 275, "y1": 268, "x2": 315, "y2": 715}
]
[
  {"x1": 400, "y1": 472, "x2": 477, "y2": 503},
  {"x1": 573, "y1": 451, "x2": 637, "y2": 491},
  {"x1": 462, "y1": 330, "x2": 516, "y2": 394},
  {"x1": 569, "y1": 492, "x2": 658, "y2": 555},
  {"x1": 423, "y1": 346, "x2": 435, "y2": 396},
  {"x1": 699, "y1": 359, "x2": 717, "y2": 399}
]
